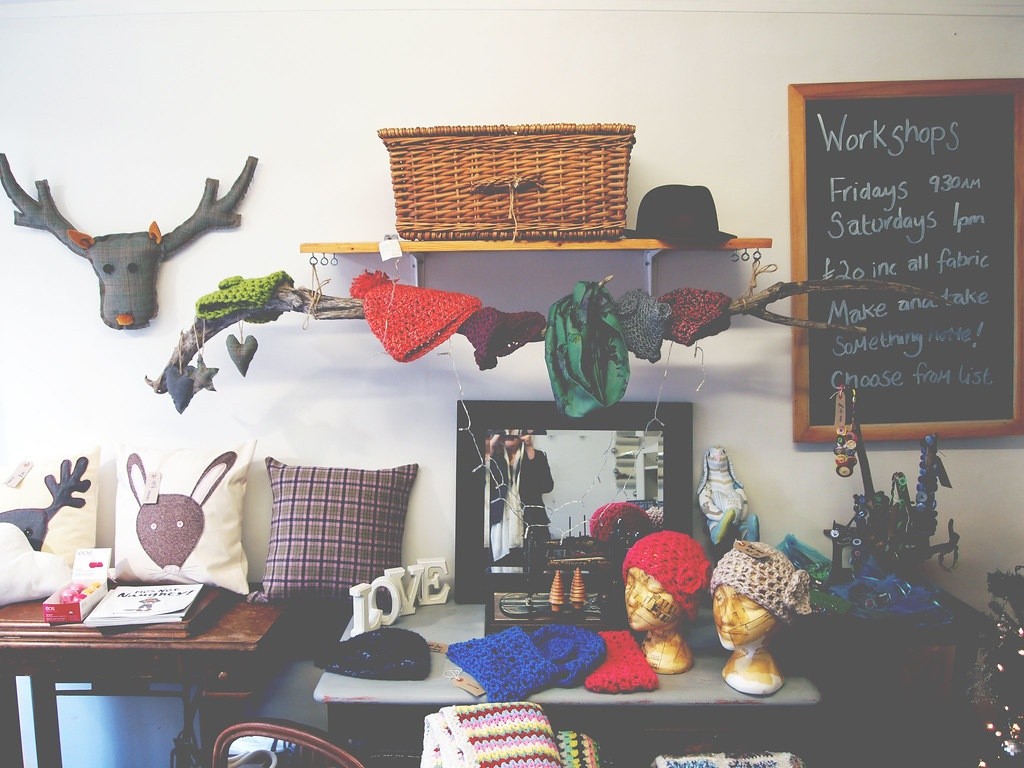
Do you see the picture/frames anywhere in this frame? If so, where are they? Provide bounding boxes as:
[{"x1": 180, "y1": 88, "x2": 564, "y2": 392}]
[{"x1": 454, "y1": 397, "x2": 695, "y2": 604}]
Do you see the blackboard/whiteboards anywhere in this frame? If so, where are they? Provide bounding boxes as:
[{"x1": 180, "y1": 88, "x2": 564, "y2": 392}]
[{"x1": 790, "y1": 79, "x2": 1024, "y2": 442}]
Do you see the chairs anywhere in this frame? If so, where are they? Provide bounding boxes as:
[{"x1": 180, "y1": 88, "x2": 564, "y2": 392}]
[{"x1": 208, "y1": 718, "x2": 364, "y2": 768}]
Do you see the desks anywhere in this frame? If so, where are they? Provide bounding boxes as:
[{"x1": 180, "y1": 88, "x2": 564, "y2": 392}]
[
  {"x1": 0, "y1": 583, "x2": 289, "y2": 768},
  {"x1": 313, "y1": 600, "x2": 821, "y2": 768}
]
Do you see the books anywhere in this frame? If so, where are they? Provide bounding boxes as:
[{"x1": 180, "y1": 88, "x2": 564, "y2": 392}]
[{"x1": 84, "y1": 584, "x2": 204, "y2": 626}]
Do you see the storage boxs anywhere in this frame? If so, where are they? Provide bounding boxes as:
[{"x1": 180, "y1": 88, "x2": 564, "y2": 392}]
[{"x1": 42, "y1": 548, "x2": 112, "y2": 624}]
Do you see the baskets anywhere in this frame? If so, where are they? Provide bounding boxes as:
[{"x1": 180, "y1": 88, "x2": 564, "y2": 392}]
[{"x1": 376, "y1": 123, "x2": 635, "y2": 240}]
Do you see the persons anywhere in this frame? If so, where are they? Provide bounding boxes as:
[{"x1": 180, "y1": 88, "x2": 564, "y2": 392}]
[
  {"x1": 623, "y1": 529, "x2": 708, "y2": 674},
  {"x1": 485, "y1": 429, "x2": 555, "y2": 566},
  {"x1": 711, "y1": 539, "x2": 811, "y2": 696}
]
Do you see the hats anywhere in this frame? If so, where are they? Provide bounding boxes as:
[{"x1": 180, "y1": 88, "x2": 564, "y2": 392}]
[
  {"x1": 659, "y1": 288, "x2": 732, "y2": 347},
  {"x1": 613, "y1": 289, "x2": 672, "y2": 363},
  {"x1": 711, "y1": 539, "x2": 812, "y2": 624},
  {"x1": 623, "y1": 530, "x2": 711, "y2": 620},
  {"x1": 589, "y1": 502, "x2": 653, "y2": 547},
  {"x1": 456, "y1": 308, "x2": 546, "y2": 371},
  {"x1": 644, "y1": 507, "x2": 663, "y2": 528},
  {"x1": 194, "y1": 270, "x2": 295, "y2": 324},
  {"x1": 349, "y1": 269, "x2": 482, "y2": 363},
  {"x1": 623, "y1": 184, "x2": 738, "y2": 246},
  {"x1": 315, "y1": 628, "x2": 430, "y2": 681}
]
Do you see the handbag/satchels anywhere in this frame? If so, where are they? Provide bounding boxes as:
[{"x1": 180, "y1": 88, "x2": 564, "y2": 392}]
[
  {"x1": 586, "y1": 631, "x2": 660, "y2": 693},
  {"x1": 446, "y1": 624, "x2": 608, "y2": 703}
]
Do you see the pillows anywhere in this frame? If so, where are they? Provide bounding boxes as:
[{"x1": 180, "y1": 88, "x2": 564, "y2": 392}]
[
  {"x1": 244, "y1": 456, "x2": 419, "y2": 606},
  {"x1": 107, "y1": 441, "x2": 250, "y2": 595},
  {"x1": 0, "y1": 446, "x2": 100, "y2": 608}
]
